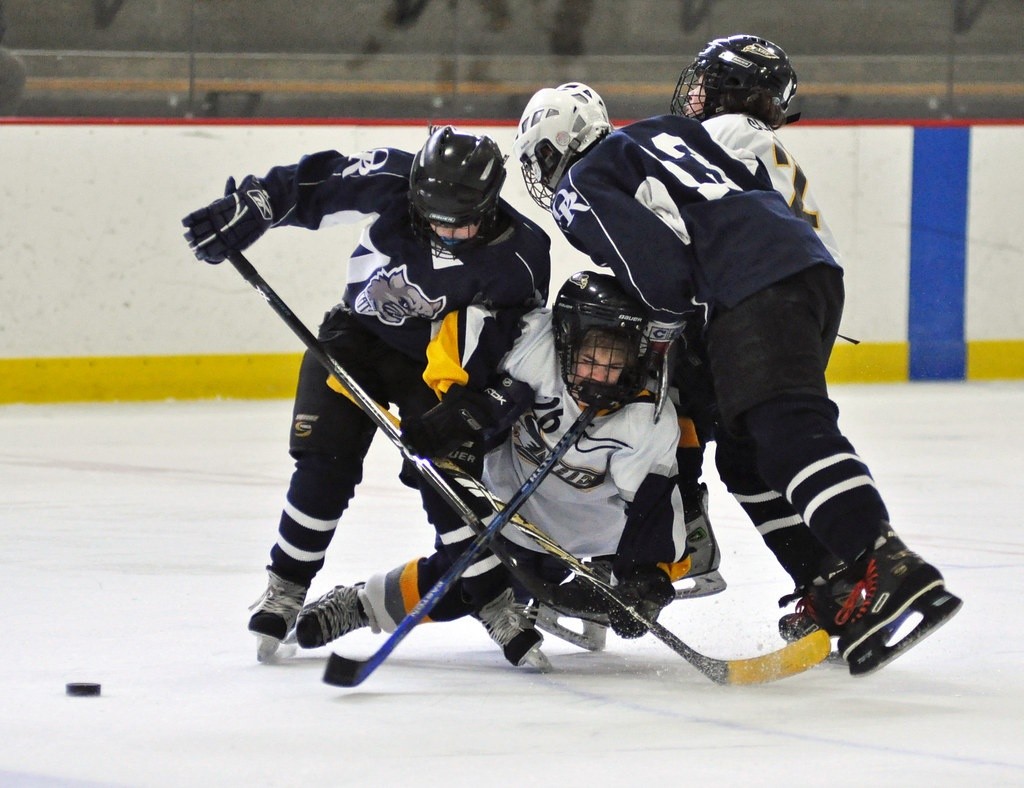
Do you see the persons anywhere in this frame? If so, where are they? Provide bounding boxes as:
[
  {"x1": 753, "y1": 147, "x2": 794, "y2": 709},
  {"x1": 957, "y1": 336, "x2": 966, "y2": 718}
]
[
  {"x1": 280, "y1": 270, "x2": 676, "y2": 655},
  {"x1": 651, "y1": 34, "x2": 841, "y2": 599},
  {"x1": 517, "y1": 83, "x2": 964, "y2": 682},
  {"x1": 179, "y1": 125, "x2": 556, "y2": 679}
]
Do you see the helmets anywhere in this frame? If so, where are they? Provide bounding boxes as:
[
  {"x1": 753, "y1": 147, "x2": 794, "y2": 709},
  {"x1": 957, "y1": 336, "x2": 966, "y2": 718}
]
[
  {"x1": 405, "y1": 120, "x2": 508, "y2": 260},
  {"x1": 515, "y1": 81, "x2": 609, "y2": 213},
  {"x1": 668, "y1": 34, "x2": 801, "y2": 128},
  {"x1": 552, "y1": 272, "x2": 633, "y2": 410}
]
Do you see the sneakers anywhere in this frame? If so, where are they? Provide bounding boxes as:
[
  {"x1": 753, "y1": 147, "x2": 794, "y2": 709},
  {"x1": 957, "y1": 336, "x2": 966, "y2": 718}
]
[
  {"x1": 475, "y1": 588, "x2": 552, "y2": 673},
  {"x1": 282, "y1": 581, "x2": 381, "y2": 649},
  {"x1": 530, "y1": 558, "x2": 613, "y2": 650},
  {"x1": 658, "y1": 484, "x2": 727, "y2": 599},
  {"x1": 778, "y1": 573, "x2": 865, "y2": 655},
  {"x1": 838, "y1": 519, "x2": 964, "y2": 677},
  {"x1": 248, "y1": 565, "x2": 312, "y2": 661}
]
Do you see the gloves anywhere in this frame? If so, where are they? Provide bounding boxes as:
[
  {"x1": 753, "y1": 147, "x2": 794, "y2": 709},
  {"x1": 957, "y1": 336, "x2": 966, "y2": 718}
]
[
  {"x1": 399, "y1": 414, "x2": 470, "y2": 460},
  {"x1": 608, "y1": 569, "x2": 677, "y2": 638},
  {"x1": 180, "y1": 175, "x2": 275, "y2": 264}
]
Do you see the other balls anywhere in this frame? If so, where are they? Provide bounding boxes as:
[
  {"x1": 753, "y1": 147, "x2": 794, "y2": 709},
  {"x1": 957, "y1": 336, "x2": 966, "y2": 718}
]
[{"x1": 64, "y1": 681, "x2": 102, "y2": 698}]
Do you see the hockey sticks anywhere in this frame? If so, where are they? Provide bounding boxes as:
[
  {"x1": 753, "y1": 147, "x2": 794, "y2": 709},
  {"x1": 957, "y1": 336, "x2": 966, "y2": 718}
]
[{"x1": 223, "y1": 247, "x2": 833, "y2": 690}]
